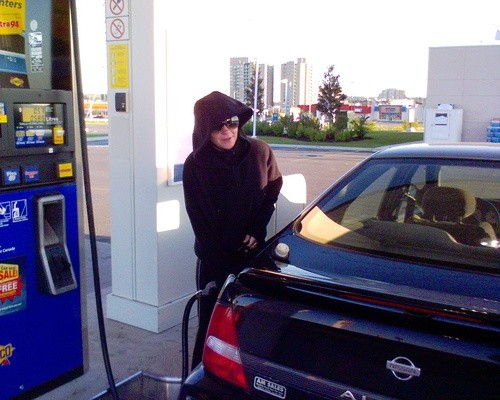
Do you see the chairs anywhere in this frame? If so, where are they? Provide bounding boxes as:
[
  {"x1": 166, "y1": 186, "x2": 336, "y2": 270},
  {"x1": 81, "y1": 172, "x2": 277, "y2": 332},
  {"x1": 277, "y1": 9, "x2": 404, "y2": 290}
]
[{"x1": 410, "y1": 186, "x2": 490, "y2": 245}]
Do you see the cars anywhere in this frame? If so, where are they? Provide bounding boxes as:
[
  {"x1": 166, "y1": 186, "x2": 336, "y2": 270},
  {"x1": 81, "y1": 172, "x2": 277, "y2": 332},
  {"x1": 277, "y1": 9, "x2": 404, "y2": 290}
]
[{"x1": 180, "y1": 141, "x2": 500, "y2": 397}]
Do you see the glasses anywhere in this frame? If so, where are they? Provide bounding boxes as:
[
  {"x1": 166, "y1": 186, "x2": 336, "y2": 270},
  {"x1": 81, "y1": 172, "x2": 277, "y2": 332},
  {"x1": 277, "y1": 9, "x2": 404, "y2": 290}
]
[{"x1": 210, "y1": 115, "x2": 242, "y2": 134}]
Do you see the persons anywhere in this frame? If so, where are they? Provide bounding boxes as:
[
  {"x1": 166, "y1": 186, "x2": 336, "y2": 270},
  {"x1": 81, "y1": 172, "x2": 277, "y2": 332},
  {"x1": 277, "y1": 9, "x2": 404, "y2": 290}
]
[{"x1": 182, "y1": 91, "x2": 283, "y2": 372}]
